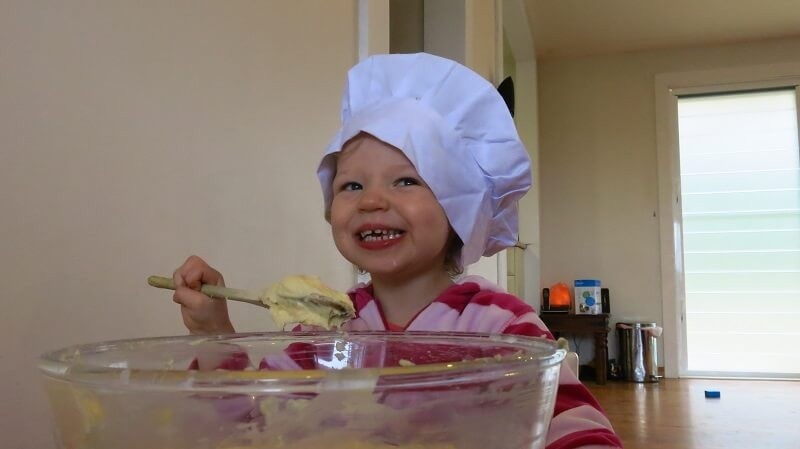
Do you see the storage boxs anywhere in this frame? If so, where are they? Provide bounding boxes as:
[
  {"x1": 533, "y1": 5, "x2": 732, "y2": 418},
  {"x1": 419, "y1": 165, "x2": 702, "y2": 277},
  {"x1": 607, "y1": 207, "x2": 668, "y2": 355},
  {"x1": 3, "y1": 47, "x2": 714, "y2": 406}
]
[{"x1": 573, "y1": 279, "x2": 603, "y2": 315}]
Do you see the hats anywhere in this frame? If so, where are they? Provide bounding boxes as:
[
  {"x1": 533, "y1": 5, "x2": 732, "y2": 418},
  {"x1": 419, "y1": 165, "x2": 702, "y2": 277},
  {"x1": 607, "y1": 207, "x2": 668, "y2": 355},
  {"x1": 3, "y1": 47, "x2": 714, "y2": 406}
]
[{"x1": 316, "y1": 51, "x2": 533, "y2": 268}]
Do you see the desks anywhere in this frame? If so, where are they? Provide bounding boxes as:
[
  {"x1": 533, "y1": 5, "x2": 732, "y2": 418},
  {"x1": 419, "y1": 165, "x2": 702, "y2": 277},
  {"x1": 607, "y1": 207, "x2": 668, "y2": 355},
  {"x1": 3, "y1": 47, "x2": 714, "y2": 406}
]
[{"x1": 539, "y1": 312, "x2": 612, "y2": 386}]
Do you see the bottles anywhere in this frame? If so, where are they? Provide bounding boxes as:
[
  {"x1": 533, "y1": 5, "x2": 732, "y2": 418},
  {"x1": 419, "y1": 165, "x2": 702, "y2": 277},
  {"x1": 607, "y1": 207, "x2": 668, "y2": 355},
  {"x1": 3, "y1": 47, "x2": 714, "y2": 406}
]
[
  {"x1": 601, "y1": 288, "x2": 610, "y2": 313},
  {"x1": 542, "y1": 288, "x2": 549, "y2": 311}
]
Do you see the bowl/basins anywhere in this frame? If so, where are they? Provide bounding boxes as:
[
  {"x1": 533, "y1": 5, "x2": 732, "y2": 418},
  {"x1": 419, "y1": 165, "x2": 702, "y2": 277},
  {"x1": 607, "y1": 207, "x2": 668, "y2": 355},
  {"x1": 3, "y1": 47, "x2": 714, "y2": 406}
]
[{"x1": 35, "y1": 332, "x2": 568, "y2": 449}]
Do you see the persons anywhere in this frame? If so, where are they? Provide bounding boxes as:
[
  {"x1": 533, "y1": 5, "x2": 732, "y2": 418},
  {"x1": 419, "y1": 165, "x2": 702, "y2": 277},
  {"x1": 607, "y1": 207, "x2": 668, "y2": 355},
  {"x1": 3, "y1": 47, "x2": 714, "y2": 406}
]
[{"x1": 173, "y1": 51, "x2": 623, "y2": 448}]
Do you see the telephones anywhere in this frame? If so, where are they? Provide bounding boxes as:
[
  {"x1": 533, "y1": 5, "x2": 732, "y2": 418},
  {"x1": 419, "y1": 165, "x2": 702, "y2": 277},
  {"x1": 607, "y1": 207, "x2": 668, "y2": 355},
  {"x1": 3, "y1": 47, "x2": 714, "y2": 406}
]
[{"x1": 540, "y1": 288, "x2": 570, "y2": 313}]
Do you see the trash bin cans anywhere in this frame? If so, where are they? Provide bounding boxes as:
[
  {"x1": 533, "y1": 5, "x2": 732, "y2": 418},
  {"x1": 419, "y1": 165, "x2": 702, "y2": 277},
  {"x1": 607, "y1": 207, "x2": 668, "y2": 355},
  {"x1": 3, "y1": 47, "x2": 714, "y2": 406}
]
[{"x1": 615, "y1": 321, "x2": 664, "y2": 382}]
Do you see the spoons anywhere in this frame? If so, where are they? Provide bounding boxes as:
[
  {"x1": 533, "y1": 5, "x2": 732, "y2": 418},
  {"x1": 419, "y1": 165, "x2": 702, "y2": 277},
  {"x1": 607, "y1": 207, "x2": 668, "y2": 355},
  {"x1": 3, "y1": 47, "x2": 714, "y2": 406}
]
[{"x1": 148, "y1": 275, "x2": 354, "y2": 322}]
